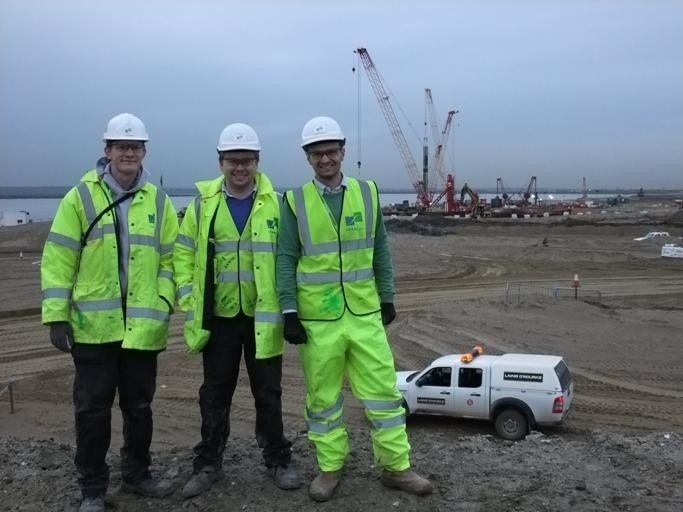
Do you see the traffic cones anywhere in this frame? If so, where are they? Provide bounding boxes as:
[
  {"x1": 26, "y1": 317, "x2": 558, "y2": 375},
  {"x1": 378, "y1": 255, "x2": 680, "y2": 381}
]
[{"x1": 571, "y1": 272, "x2": 582, "y2": 288}]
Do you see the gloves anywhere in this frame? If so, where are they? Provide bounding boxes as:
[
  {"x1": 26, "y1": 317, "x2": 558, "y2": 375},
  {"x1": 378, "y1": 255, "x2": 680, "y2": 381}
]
[
  {"x1": 284, "y1": 313, "x2": 307, "y2": 344},
  {"x1": 49, "y1": 321, "x2": 75, "y2": 353},
  {"x1": 380, "y1": 302, "x2": 396, "y2": 325}
]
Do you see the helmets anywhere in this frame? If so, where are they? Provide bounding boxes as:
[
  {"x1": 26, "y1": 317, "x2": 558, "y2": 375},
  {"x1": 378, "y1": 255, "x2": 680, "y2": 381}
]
[
  {"x1": 301, "y1": 117, "x2": 346, "y2": 149},
  {"x1": 102, "y1": 113, "x2": 152, "y2": 143},
  {"x1": 216, "y1": 123, "x2": 261, "y2": 154}
]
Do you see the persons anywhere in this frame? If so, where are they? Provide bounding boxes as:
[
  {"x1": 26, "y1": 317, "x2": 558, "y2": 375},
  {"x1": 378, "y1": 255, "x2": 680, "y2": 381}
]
[
  {"x1": 274, "y1": 118, "x2": 433, "y2": 503},
  {"x1": 39, "y1": 111, "x2": 181, "y2": 511},
  {"x1": 175, "y1": 122, "x2": 300, "y2": 500}
]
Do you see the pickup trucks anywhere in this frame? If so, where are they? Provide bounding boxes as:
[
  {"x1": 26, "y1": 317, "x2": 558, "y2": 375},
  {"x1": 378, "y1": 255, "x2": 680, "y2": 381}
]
[
  {"x1": 634, "y1": 231, "x2": 683, "y2": 243},
  {"x1": 394, "y1": 345, "x2": 575, "y2": 440}
]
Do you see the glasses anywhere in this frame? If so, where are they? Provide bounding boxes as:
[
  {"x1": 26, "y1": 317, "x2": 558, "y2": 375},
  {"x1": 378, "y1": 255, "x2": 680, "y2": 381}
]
[
  {"x1": 109, "y1": 142, "x2": 146, "y2": 152},
  {"x1": 220, "y1": 157, "x2": 257, "y2": 167},
  {"x1": 304, "y1": 148, "x2": 342, "y2": 160}
]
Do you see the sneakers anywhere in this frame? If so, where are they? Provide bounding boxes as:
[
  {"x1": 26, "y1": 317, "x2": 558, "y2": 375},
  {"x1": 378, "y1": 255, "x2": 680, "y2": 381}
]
[
  {"x1": 79, "y1": 495, "x2": 104, "y2": 512},
  {"x1": 309, "y1": 469, "x2": 345, "y2": 502},
  {"x1": 381, "y1": 467, "x2": 433, "y2": 496},
  {"x1": 258, "y1": 461, "x2": 301, "y2": 490},
  {"x1": 120, "y1": 478, "x2": 176, "y2": 499},
  {"x1": 181, "y1": 463, "x2": 224, "y2": 498}
]
